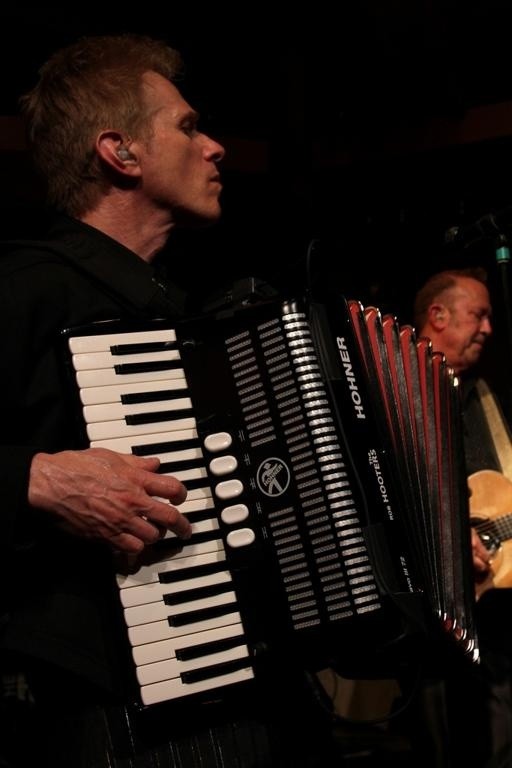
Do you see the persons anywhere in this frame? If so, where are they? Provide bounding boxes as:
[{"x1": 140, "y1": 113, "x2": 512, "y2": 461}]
[
  {"x1": 307, "y1": 266, "x2": 492, "y2": 764},
  {"x1": 4, "y1": 29, "x2": 229, "y2": 758}
]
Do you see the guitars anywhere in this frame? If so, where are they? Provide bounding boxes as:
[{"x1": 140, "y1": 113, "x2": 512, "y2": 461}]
[{"x1": 466, "y1": 469, "x2": 512, "y2": 601}]
